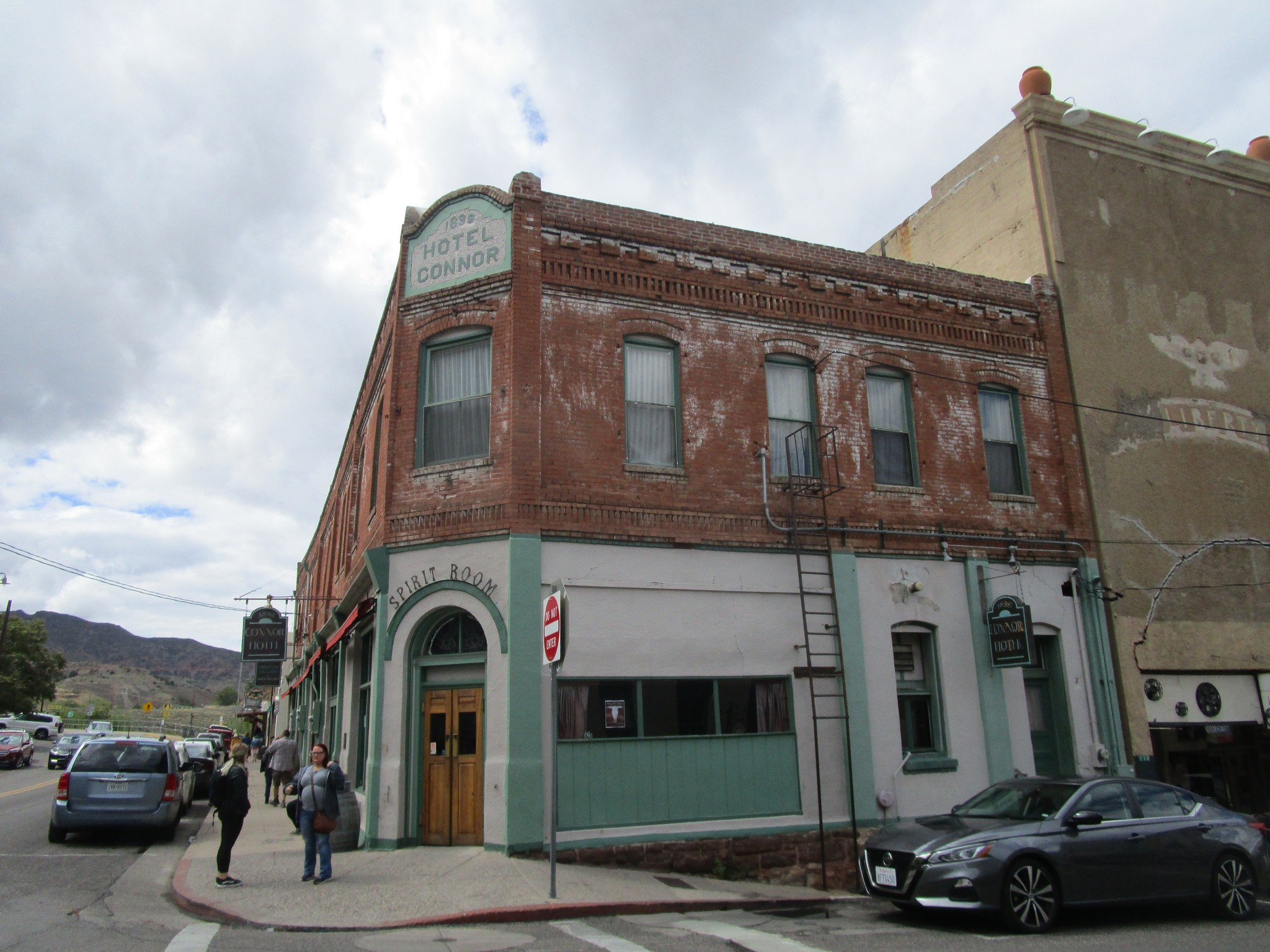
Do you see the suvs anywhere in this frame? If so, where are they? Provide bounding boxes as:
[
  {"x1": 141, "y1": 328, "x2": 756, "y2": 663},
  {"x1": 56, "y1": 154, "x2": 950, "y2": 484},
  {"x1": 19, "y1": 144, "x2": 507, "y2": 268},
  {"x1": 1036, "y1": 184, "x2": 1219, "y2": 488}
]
[{"x1": 0, "y1": 712, "x2": 65, "y2": 740}]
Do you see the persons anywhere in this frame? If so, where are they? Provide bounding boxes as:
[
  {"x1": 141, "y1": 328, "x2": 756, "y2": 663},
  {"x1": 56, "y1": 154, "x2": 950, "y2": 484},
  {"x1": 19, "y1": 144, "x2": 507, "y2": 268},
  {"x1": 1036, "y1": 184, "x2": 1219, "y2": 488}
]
[
  {"x1": 260, "y1": 730, "x2": 299, "y2": 808},
  {"x1": 231, "y1": 726, "x2": 263, "y2": 762},
  {"x1": 282, "y1": 744, "x2": 345, "y2": 885},
  {"x1": 207, "y1": 743, "x2": 251, "y2": 889}
]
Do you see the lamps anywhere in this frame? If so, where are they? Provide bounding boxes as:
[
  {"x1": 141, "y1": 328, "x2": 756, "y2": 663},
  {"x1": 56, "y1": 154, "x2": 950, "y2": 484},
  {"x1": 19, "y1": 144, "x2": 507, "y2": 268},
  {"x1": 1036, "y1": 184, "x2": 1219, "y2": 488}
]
[
  {"x1": 1204, "y1": 139, "x2": 1232, "y2": 163},
  {"x1": 1009, "y1": 545, "x2": 1017, "y2": 566},
  {"x1": 908, "y1": 581, "x2": 925, "y2": 593},
  {"x1": 303, "y1": 562, "x2": 309, "y2": 571},
  {"x1": 1135, "y1": 118, "x2": 1161, "y2": 142},
  {"x1": 1060, "y1": 97, "x2": 1090, "y2": 124},
  {"x1": 941, "y1": 542, "x2": 952, "y2": 562},
  {"x1": 293, "y1": 591, "x2": 296, "y2": 596}
]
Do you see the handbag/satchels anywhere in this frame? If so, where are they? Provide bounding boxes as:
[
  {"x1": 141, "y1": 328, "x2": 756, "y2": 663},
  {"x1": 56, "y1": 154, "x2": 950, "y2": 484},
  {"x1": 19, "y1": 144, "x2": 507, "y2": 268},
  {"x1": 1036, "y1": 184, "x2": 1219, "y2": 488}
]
[{"x1": 313, "y1": 813, "x2": 337, "y2": 834}]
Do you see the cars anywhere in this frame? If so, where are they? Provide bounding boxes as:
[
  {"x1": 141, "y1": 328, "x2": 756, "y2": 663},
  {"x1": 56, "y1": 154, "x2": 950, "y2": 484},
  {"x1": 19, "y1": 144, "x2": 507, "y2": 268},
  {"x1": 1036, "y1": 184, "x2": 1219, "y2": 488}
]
[
  {"x1": 85, "y1": 720, "x2": 114, "y2": 737},
  {"x1": 48, "y1": 733, "x2": 193, "y2": 843},
  {"x1": 117, "y1": 724, "x2": 233, "y2": 816},
  {"x1": 0, "y1": 729, "x2": 35, "y2": 769},
  {"x1": 47, "y1": 734, "x2": 99, "y2": 770},
  {"x1": 858, "y1": 775, "x2": 1270, "y2": 935}
]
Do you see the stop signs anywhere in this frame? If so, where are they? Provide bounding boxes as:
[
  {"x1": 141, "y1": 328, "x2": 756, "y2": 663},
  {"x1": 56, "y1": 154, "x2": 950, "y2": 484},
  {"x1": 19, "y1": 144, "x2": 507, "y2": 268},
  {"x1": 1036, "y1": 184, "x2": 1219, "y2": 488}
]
[{"x1": 540, "y1": 590, "x2": 562, "y2": 665}]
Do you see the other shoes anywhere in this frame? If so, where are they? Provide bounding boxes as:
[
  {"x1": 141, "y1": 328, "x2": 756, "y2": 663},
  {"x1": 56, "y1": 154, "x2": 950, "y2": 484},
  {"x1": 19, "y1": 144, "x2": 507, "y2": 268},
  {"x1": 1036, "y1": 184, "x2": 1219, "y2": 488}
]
[
  {"x1": 269, "y1": 799, "x2": 277, "y2": 807},
  {"x1": 313, "y1": 876, "x2": 332, "y2": 885},
  {"x1": 215, "y1": 876, "x2": 243, "y2": 889},
  {"x1": 301, "y1": 872, "x2": 315, "y2": 881},
  {"x1": 281, "y1": 800, "x2": 286, "y2": 808}
]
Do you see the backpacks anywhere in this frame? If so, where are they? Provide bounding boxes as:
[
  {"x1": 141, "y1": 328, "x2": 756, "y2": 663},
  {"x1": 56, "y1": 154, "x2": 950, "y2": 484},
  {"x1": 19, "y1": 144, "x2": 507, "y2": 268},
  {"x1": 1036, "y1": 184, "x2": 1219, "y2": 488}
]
[{"x1": 209, "y1": 770, "x2": 234, "y2": 810}]
